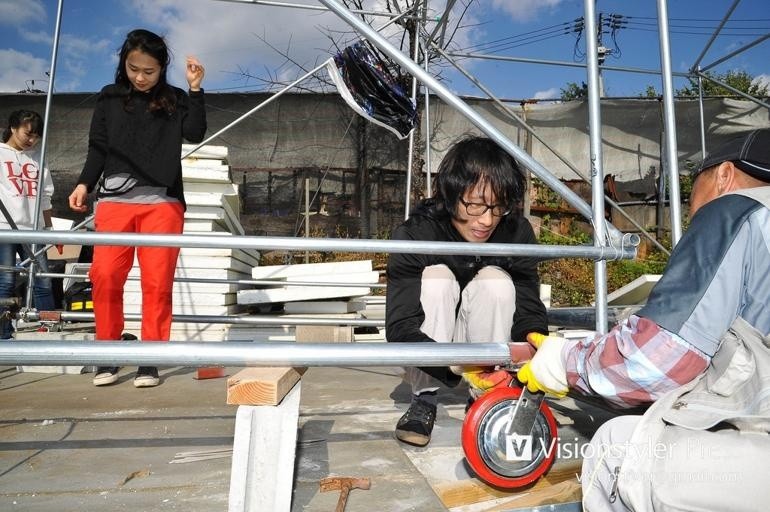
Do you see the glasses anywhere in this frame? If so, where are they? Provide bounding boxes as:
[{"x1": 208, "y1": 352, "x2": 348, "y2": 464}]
[{"x1": 458, "y1": 196, "x2": 512, "y2": 217}]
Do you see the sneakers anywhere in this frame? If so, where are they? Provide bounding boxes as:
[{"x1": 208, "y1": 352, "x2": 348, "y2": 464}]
[
  {"x1": 93, "y1": 368, "x2": 118, "y2": 386},
  {"x1": 134, "y1": 366, "x2": 159, "y2": 388},
  {"x1": 395, "y1": 396, "x2": 437, "y2": 447},
  {"x1": 1, "y1": 323, "x2": 14, "y2": 339},
  {"x1": 464, "y1": 394, "x2": 476, "y2": 414}
]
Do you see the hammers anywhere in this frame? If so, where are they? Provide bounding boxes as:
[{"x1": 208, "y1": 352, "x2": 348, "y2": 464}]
[{"x1": 319, "y1": 477, "x2": 371, "y2": 512}]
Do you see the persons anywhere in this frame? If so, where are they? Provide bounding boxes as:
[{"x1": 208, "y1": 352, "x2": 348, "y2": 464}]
[
  {"x1": 385, "y1": 134, "x2": 550, "y2": 447},
  {"x1": 516, "y1": 127, "x2": 770, "y2": 512},
  {"x1": 0, "y1": 107, "x2": 65, "y2": 338},
  {"x1": 67, "y1": 27, "x2": 209, "y2": 387}
]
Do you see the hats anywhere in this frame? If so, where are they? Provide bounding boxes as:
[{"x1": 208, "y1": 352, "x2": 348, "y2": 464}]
[{"x1": 692, "y1": 127, "x2": 770, "y2": 182}]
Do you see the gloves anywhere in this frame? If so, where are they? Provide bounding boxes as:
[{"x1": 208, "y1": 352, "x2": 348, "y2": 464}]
[
  {"x1": 449, "y1": 365, "x2": 512, "y2": 401},
  {"x1": 516, "y1": 332, "x2": 570, "y2": 399}
]
